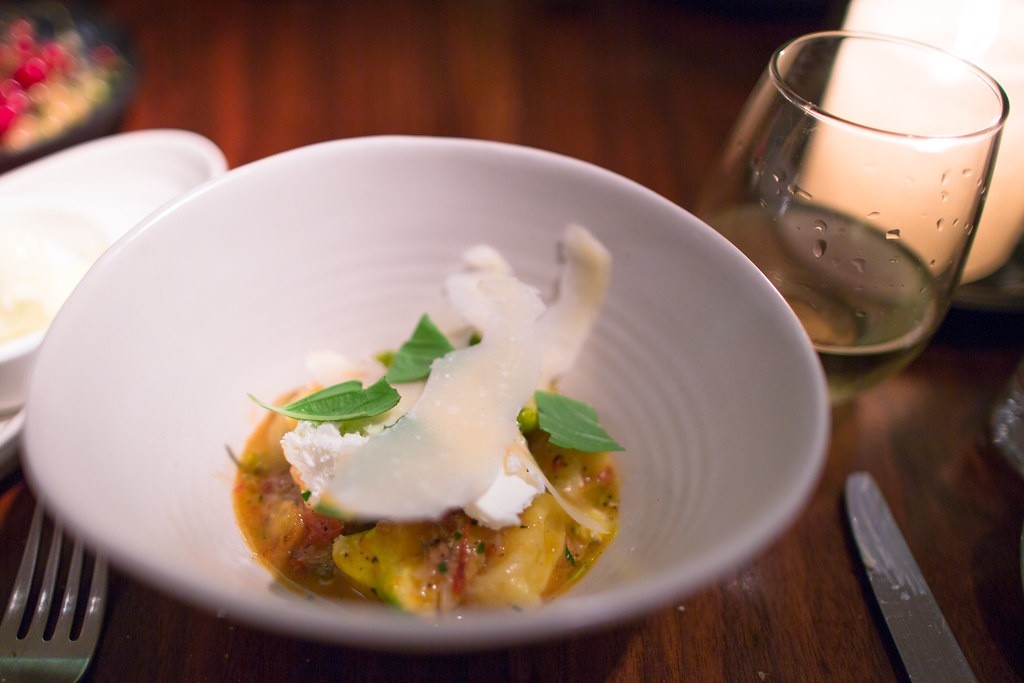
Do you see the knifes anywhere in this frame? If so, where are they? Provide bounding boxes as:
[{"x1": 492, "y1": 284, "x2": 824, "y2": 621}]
[{"x1": 846, "y1": 472, "x2": 977, "y2": 683}]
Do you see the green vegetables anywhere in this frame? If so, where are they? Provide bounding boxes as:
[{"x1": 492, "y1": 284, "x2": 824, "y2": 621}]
[{"x1": 243, "y1": 312, "x2": 627, "y2": 451}]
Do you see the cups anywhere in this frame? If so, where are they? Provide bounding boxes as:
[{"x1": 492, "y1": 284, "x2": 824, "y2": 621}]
[{"x1": 699, "y1": 29, "x2": 1010, "y2": 409}]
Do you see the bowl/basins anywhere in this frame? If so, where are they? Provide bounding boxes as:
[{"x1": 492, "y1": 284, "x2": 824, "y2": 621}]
[{"x1": 22, "y1": 136, "x2": 832, "y2": 654}]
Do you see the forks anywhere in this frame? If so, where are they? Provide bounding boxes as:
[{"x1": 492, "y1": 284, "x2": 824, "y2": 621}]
[{"x1": 0, "y1": 498, "x2": 107, "y2": 683}]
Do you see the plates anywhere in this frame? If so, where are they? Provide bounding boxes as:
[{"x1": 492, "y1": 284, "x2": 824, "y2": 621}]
[
  {"x1": 0, "y1": 14, "x2": 141, "y2": 164},
  {"x1": 0, "y1": 128, "x2": 231, "y2": 474}
]
